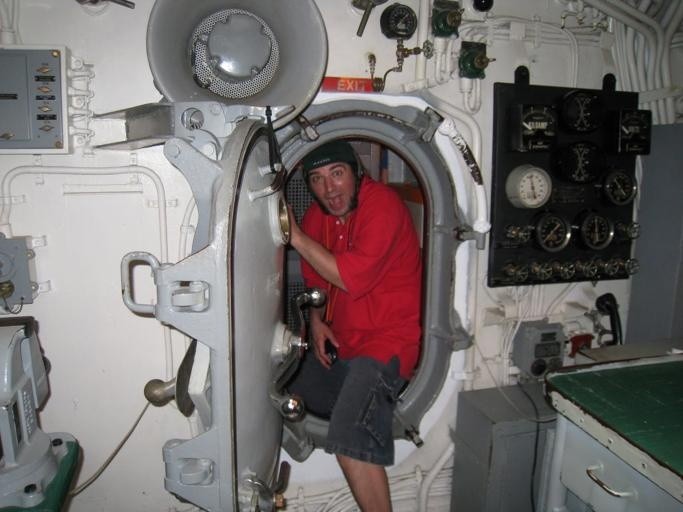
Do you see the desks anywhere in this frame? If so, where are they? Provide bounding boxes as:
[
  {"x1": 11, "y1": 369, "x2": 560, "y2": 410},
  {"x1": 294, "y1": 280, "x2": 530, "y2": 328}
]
[{"x1": 538, "y1": 352, "x2": 682, "y2": 511}]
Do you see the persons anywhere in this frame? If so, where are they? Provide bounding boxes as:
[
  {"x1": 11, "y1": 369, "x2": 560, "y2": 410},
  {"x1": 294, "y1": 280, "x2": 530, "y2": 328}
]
[{"x1": 286, "y1": 141, "x2": 423, "y2": 512}]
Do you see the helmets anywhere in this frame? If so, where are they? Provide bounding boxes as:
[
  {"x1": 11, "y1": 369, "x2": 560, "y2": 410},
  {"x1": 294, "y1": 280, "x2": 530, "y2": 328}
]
[{"x1": 302, "y1": 141, "x2": 364, "y2": 182}]
